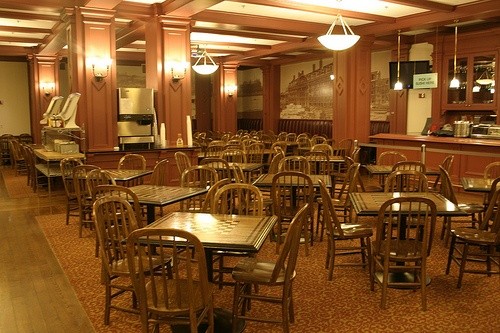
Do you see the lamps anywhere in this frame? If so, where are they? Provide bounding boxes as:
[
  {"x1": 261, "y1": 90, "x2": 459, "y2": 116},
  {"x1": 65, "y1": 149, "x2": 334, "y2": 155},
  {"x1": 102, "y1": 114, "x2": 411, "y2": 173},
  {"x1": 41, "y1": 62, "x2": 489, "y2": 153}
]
[
  {"x1": 192, "y1": 41, "x2": 219, "y2": 74},
  {"x1": 89, "y1": 56, "x2": 112, "y2": 80},
  {"x1": 40, "y1": 82, "x2": 55, "y2": 95},
  {"x1": 317, "y1": 0, "x2": 360, "y2": 51},
  {"x1": 394, "y1": 30, "x2": 403, "y2": 90},
  {"x1": 450, "y1": 20, "x2": 461, "y2": 87},
  {"x1": 169, "y1": 62, "x2": 190, "y2": 80}
]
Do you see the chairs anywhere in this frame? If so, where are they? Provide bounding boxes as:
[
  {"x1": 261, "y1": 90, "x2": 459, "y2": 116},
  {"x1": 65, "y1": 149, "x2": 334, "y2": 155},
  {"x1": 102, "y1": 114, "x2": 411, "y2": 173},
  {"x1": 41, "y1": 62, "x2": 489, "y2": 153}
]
[{"x1": 0, "y1": 133, "x2": 500, "y2": 333}]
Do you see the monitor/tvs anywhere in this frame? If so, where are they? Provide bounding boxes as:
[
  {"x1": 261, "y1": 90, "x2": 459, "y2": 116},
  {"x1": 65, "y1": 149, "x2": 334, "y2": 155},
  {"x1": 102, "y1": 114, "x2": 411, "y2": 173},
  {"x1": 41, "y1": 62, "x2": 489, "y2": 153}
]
[{"x1": 389, "y1": 61, "x2": 430, "y2": 89}]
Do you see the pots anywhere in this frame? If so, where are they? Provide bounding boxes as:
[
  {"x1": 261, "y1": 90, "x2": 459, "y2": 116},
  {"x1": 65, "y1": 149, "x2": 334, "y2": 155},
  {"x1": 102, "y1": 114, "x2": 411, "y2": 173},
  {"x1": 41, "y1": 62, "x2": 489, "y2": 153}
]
[{"x1": 453, "y1": 120, "x2": 471, "y2": 137}]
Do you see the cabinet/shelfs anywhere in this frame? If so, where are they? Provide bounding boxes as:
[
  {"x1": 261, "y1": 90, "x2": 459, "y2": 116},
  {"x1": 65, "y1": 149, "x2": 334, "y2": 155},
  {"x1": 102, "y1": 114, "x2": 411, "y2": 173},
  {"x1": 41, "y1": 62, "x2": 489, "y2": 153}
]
[
  {"x1": 34, "y1": 124, "x2": 86, "y2": 198},
  {"x1": 442, "y1": 48, "x2": 500, "y2": 116}
]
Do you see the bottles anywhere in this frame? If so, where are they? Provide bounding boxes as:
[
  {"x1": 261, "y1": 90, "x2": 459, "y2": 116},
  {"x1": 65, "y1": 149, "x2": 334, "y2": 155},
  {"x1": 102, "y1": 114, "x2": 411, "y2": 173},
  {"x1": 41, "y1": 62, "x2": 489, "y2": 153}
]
[{"x1": 176, "y1": 134, "x2": 183, "y2": 147}]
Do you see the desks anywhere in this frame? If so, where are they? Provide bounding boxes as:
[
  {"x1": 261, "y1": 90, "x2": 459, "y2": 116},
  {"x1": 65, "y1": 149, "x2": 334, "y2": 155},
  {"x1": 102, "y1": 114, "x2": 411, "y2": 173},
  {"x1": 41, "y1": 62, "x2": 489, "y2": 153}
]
[
  {"x1": 84, "y1": 185, "x2": 208, "y2": 256},
  {"x1": 362, "y1": 165, "x2": 441, "y2": 191},
  {"x1": 346, "y1": 191, "x2": 469, "y2": 288},
  {"x1": 253, "y1": 174, "x2": 332, "y2": 208},
  {"x1": 118, "y1": 211, "x2": 278, "y2": 333},
  {"x1": 200, "y1": 163, "x2": 267, "y2": 214},
  {"x1": 66, "y1": 169, "x2": 154, "y2": 191},
  {"x1": 289, "y1": 155, "x2": 345, "y2": 175}
]
